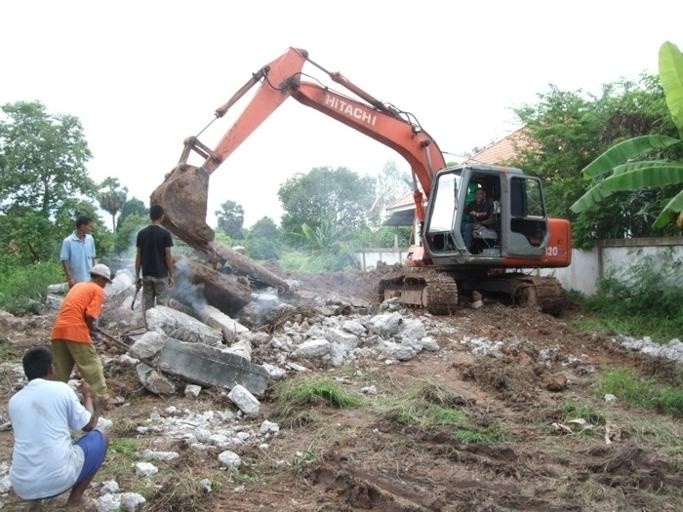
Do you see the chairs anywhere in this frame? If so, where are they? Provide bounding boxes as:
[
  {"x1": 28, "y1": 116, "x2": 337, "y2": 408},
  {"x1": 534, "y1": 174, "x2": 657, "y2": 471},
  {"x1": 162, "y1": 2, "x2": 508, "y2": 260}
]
[{"x1": 472, "y1": 202, "x2": 498, "y2": 253}]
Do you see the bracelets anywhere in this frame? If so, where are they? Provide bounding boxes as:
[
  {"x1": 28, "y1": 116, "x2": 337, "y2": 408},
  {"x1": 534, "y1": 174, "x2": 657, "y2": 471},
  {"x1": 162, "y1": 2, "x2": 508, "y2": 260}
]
[
  {"x1": 84, "y1": 393, "x2": 92, "y2": 400},
  {"x1": 66, "y1": 276, "x2": 73, "y2": 283},
  {"x1": 475, "y1": 211, "x2": 479, "y2": 217}
]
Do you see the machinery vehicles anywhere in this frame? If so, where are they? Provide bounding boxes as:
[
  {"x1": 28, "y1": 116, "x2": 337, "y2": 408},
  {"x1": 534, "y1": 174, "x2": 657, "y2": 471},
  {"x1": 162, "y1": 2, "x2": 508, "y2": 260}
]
[{"x1": 150, "y1": 47, "x2": 572, "y2": 315}]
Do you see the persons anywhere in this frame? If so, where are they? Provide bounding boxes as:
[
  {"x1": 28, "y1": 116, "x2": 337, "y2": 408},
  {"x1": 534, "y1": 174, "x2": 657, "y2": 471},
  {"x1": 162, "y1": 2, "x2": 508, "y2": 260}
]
[
  {"x1": 129, "y1": 204, "x2": 174, "y2": 332},
  {"x1": 57, "y1": 213, "x2": 104, "y2": 344},
  {"x1": 46, "y1": 264, "x2": 121, "y2": 412},
  {"x1": 5, "y1": 345, "x2": 110, "y2": 511},
  {"x1": 457, "y1": 187, "x2": 493, "y2": 254}
]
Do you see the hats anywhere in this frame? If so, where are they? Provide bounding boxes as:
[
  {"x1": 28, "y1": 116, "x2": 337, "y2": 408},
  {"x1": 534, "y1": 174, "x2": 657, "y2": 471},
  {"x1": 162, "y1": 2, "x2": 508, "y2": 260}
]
[{"x1": 88, "y1": 264, "x2": 112, "y2": 284}]
